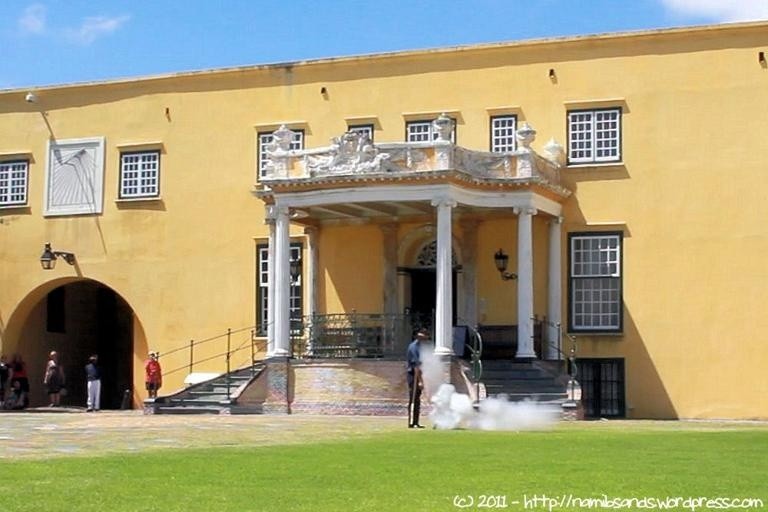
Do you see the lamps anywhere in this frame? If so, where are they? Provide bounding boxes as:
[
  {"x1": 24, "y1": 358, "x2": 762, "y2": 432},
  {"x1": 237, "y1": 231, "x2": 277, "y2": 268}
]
[
  {"x1": 287, "y1": 256, "x2": 301, "y2": 284},
  {"x1": 38, "y1": 241, "x2": 75, "y2": 271},
  {"x1": 164, "y1": 108, "x2": 171, "y2": 118},
  {"x1": 492, "y1": 248, "x2": 519, "y2": 281},
  {"x1": 759, "y1": 51, "x2": 765, "y2": 65},
  {"x1": 549, "y1": 69, "x2": 556, "y2": 80},
  {"x1": 321, "y1": 88, "x2": 327, "y2": 95}
]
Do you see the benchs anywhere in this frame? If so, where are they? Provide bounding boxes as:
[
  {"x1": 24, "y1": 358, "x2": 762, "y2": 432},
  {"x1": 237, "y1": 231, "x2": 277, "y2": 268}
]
[{"x1": 469, "y1": 320, "x2": 543, "y2": 359}]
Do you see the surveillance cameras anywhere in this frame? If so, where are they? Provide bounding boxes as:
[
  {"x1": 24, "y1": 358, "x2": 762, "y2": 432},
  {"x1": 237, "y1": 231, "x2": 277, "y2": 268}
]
[{"x1": 25, "y1": 94, "x2": 34, "y2": 103}]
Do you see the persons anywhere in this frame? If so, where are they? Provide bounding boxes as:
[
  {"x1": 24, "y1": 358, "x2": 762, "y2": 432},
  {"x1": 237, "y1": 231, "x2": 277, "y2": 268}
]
[
  {"x1": 42, "y1": 350, "x2": 66, "y2": 407},
  {"x1": 405, "y1": 328, "x2": 429, "y2": 429},
  {"x1": 143, "y1": 350, "x2": 163, "y2": 398},
  {"x1": 84, "y1": 354, "x2": 102, "y2": 412},
  {"x1": 1, "y1": 353, "x2": 30, "y2": 411}
]
